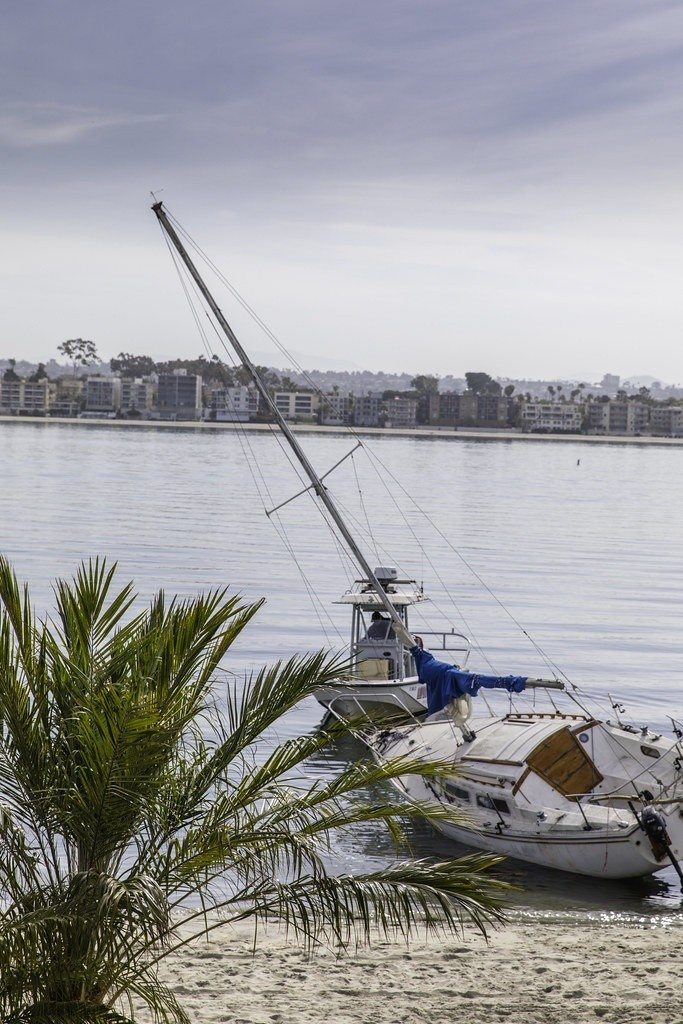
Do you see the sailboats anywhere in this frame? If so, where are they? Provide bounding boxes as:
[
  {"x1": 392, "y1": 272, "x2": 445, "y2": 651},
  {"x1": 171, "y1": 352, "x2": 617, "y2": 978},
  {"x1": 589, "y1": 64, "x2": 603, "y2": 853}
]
[{"x1": 147, "y1": 189, "x2": 683, "y2": 894}]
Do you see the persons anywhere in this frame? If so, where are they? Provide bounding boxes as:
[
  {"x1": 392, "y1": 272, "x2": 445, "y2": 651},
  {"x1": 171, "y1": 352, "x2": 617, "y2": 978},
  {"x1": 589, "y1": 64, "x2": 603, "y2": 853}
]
[{"x1": 363, "y1": 611, "x2": 395, "y2": 639}]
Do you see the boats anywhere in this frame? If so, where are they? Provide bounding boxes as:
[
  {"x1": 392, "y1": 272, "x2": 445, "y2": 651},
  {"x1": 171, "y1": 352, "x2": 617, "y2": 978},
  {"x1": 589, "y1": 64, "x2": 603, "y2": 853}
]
[{"x1": 306, "y1": 564, "x2": 475, "y2": 729}]
